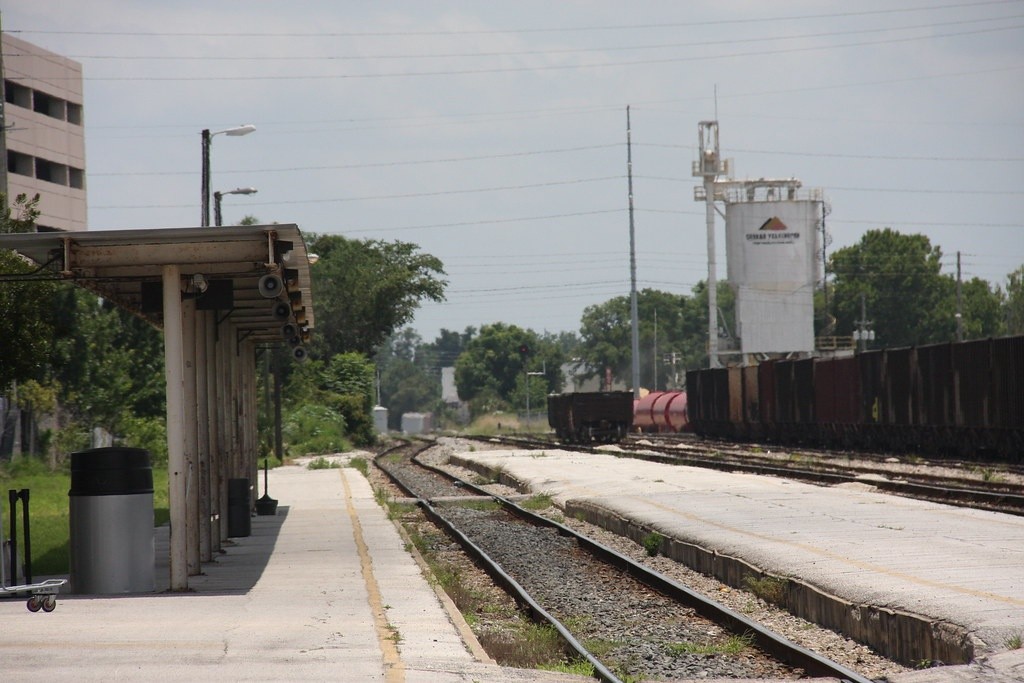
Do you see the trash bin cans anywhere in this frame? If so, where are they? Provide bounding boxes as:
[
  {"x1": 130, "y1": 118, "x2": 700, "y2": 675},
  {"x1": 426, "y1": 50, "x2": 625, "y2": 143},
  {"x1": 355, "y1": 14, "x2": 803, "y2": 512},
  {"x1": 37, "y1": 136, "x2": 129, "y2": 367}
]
[
  {"x1": 228, "y1": 478, "x2": 250, "y2": 537},
  {"x1": 70, "y1": 447, "x2": 156, "y2": 594}
]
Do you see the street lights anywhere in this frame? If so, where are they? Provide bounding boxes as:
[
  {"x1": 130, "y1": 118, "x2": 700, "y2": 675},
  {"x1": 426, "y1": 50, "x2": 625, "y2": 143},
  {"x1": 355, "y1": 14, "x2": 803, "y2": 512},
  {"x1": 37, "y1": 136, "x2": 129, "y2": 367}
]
[
  {"x1": 197, "y1": 123, "x2": 254, "y2": 226},
  {"x1": 213, "y1": 183, "x2": 259, "y2": 228}
]
